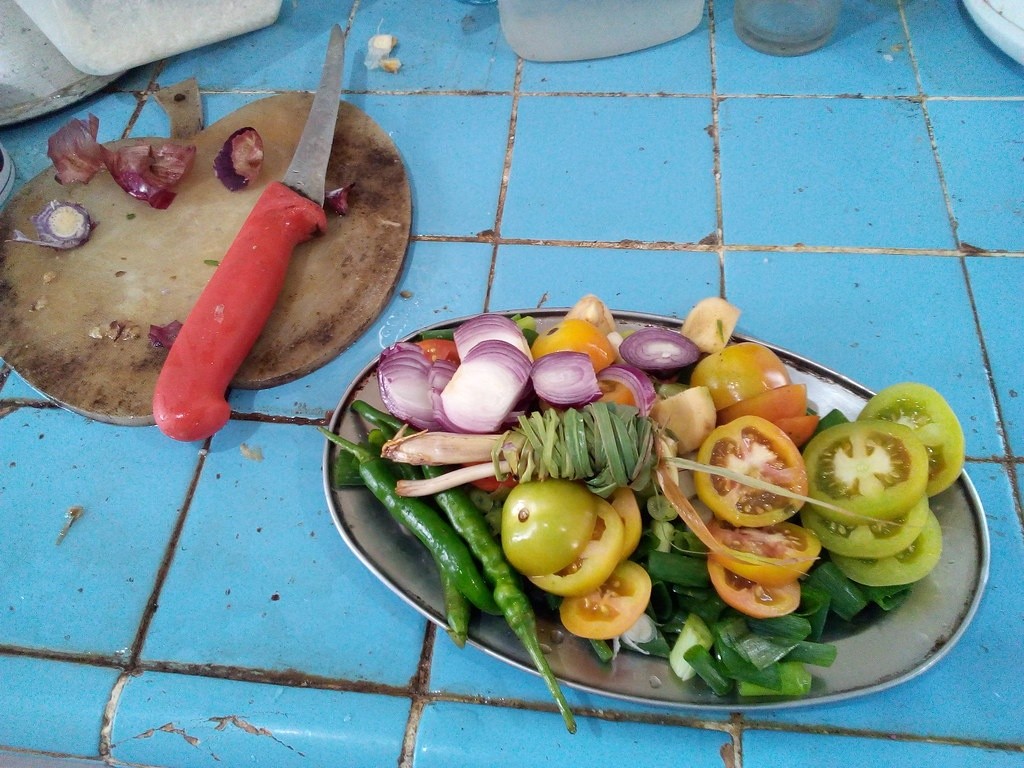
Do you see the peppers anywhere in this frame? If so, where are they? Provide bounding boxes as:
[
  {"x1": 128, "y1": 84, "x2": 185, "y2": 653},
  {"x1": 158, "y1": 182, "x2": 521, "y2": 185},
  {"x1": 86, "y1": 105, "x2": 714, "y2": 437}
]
[{"x1": 318, "y1": 401, "x2": 577, "y2": 732}]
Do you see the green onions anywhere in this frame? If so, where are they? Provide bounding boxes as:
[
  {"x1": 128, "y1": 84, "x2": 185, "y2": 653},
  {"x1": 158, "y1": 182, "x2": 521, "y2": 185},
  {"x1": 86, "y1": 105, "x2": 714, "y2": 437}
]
[{"x1": 469, "y1": 480, "x2": 913, "y2": 699}]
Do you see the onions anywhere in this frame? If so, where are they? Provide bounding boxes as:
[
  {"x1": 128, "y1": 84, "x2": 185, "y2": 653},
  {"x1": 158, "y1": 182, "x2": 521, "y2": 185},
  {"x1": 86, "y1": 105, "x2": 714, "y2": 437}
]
[{"x1": 378, "y1": 311, "x2": 704, "y2": 434}]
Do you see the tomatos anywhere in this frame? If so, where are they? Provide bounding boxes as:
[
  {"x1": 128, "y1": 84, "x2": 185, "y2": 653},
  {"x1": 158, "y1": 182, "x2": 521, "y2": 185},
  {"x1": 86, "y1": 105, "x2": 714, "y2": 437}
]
[{"x1": 411, "y1": 320, "x2": 971, "y2": 639}]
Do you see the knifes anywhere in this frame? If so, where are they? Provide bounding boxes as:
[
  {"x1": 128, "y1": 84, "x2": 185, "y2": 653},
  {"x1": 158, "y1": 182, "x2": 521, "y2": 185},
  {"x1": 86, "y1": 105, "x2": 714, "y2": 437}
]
[{"x1": 151, "y1": 24, "x2": 346, "y2": 441}]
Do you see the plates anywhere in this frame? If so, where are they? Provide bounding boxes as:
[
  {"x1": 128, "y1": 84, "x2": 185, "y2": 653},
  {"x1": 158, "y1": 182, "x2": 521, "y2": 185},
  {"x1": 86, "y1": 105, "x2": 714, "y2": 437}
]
[{"x1": 324, "y1": 309, "x2": 991, "y2": 712}]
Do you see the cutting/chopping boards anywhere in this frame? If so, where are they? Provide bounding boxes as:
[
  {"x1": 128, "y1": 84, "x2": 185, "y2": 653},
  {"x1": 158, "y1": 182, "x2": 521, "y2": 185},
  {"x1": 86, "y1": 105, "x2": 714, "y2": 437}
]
[{"x1": 1, "y1": 80, "x2": 411, "y2": 428}]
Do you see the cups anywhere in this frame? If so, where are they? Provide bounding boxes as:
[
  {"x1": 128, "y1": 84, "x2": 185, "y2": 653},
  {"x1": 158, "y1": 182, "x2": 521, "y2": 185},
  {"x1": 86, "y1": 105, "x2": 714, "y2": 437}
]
[{"x1": 733, "y1": 0, "x2": 836, "y2": 58}]
